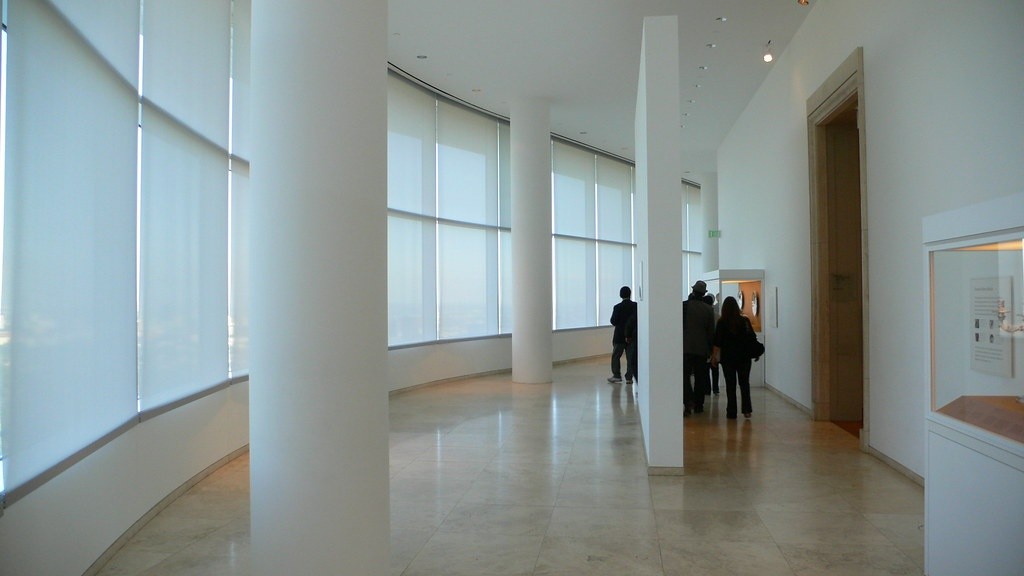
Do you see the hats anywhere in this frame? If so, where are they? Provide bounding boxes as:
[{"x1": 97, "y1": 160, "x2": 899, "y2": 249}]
[{"x1": 692, "y1": 281, "x2": 707, "y2": 293}]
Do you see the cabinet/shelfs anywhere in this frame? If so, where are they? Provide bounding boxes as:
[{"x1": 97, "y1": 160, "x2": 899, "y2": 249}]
[
  {"x1": 915, "y1": 183, "x2": 1024, "y2": 574},
  {"x1": 698, "y1": 267, "x2": 767, "y2": 388}
]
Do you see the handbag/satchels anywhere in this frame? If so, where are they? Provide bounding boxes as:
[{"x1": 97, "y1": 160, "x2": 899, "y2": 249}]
[{"x1": 746, "y1": 318, "x2": 765, "y2": 358}]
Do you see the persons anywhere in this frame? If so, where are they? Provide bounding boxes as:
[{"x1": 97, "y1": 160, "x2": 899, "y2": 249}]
[
  {"x1": 711, "y1": 296, "x2": 761, "y2": 419},
  {"x1": 625, "y1": 279, "x2": 721, "y2": 416},
  {"x1": 607, "y1": 286, "x2": 635, "y2": 383}
]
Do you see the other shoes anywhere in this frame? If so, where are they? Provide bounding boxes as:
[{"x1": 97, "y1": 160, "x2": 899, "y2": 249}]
[
  {"x1": 625, "y1": 380, "x2": 633, "y2": 384},
  {"x1": 607, "y1": 377, "x2": 622, "y2": 383},
  {"x1": 707, "y1": 391, "x2": 720, "y2": 395},
  {"x1": 745, "y1": 413, "x2": 751, "y2": 418},
  {"x1": 684, "y1": 408, "x2": 704, "y2": 417}
]
